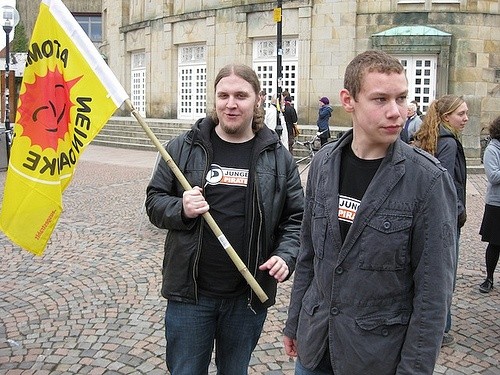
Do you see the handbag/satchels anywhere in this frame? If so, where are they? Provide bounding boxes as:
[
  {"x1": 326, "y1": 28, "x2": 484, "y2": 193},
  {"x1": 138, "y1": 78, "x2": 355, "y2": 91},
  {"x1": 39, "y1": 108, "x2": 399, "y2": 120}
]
[{"x1": 292, "y1": 124, "x2": 300, "y2": 136}]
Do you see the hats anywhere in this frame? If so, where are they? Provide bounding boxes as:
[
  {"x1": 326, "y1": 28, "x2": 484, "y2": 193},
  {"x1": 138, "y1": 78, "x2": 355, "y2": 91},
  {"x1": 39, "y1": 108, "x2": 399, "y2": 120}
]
[{"x1": 319, "y1": 97, "x2": 329, "y2": 104}]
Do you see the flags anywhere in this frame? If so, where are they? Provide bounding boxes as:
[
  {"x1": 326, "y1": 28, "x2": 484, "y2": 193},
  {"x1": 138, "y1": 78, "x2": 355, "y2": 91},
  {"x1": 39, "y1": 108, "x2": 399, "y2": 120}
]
[{"x1": 0, "y1": 0, "x2": 129, "y2": 258}]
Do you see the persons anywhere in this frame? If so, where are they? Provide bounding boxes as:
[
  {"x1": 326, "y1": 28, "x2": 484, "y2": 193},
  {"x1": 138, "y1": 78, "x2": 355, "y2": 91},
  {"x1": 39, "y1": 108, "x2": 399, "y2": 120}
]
[
  {"x1": 478, "y1": 116, "x2": 500, "y2": 294},
  {"x1": 413, "y1": 95, "x2": 469, "y2": 347},
  {"x1": 258, "y1": 91, "x2": 425, "y2": 153},
  {"x1": 283, "y1": 49, "x2": 457, "y2": 375},
  {"x1": 145, "y1": 63, "x2": 305, "y2": 375}
]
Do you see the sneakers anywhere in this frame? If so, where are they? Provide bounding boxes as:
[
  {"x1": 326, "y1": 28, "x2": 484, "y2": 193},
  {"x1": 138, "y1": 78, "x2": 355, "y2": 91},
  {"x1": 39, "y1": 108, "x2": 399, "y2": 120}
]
[
  {"x1": 441, "y1": 333, "x2": 455, "y2": 347},
  {"x1": 479, "y1": 277, "x2": 493, "y2": 293}
]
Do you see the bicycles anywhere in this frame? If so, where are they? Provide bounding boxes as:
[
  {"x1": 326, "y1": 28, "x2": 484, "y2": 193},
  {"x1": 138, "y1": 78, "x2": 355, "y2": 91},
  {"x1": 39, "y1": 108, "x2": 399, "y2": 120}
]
[{"x1": 288, "y1": 128, "x2": 329, "y2": 176}]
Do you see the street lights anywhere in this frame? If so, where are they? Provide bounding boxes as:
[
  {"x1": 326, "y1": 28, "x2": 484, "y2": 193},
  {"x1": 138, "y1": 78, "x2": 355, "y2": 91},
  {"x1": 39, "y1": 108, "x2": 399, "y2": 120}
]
[{"x1": 1, "y1": 5, "x2": 20, "y2": 71}]
